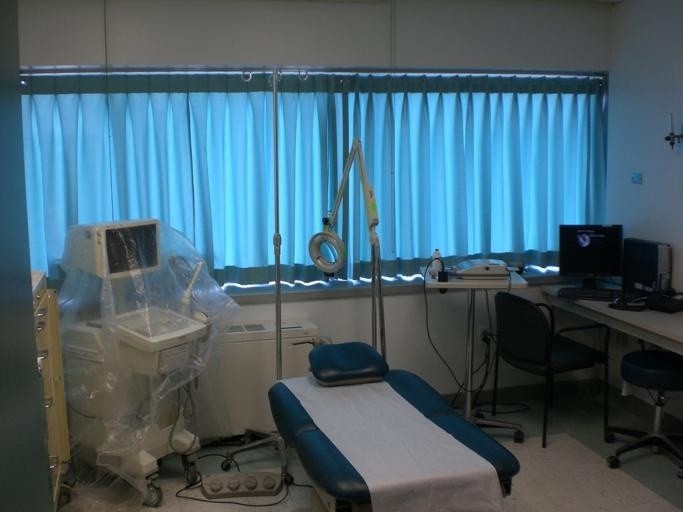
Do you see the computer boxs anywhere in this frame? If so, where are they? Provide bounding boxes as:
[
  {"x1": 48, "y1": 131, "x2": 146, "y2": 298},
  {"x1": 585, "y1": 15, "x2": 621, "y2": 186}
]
[{"x1": 622, "y1": 238, "x2": 672, "y2": 302}]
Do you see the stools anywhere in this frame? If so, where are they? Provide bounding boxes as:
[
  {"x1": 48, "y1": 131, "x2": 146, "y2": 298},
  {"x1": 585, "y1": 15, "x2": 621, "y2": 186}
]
[{"x1": 607, "y1": 349, "x2": 682, "y2": 479}]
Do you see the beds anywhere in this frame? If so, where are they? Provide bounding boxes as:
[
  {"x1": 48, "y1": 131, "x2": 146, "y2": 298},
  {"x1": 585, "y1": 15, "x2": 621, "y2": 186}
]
[{"x1": 268, "y1": 367, "x2": 521, "y2": 512}]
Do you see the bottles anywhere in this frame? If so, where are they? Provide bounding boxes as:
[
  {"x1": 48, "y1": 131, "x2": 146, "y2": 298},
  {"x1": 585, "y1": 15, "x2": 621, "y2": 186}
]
[{"x1": 431, "y1": 249, "x2": 443, "y2": 276}]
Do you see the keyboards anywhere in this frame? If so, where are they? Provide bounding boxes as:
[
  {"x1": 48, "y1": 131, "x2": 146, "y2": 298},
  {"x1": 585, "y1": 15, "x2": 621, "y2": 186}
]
[{"x1": 558, "y1": 287, "x2": 622, "y2": 300}]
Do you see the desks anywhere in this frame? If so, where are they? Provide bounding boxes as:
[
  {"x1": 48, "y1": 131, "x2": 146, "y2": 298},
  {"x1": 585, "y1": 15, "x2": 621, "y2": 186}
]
[
  {"x1": 421, "y1": 267, "x2": 528, "y2": 443},
  {"x1": 541, "y1": 284, "x2": 683, "y2": 356}
]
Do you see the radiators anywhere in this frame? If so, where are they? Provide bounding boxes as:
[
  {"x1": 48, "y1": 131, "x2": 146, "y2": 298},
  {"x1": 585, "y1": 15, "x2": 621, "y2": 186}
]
[{"x1": 198, "y1": 322, "x2": 320, "y2": 438}]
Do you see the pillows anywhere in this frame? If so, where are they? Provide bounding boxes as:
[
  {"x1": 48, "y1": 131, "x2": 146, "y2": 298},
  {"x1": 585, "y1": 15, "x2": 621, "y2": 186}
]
[{"x1": 308, "y1": 341, "x2": 388, "y2": 387}]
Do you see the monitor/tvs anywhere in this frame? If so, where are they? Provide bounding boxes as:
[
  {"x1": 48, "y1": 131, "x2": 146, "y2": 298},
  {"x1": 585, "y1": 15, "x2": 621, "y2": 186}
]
[
  {"x1": 559, "y1": 225, "x2": 623, "y2": 276},
  {"x1": 70, "y1": 219, "x2": 162, "y2": 280}
]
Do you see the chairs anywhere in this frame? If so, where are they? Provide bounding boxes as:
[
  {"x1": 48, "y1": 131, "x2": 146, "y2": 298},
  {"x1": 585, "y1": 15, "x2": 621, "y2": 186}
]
[{"x1": 492, "y1": 290, "x2": 610, "y2": 449}]
